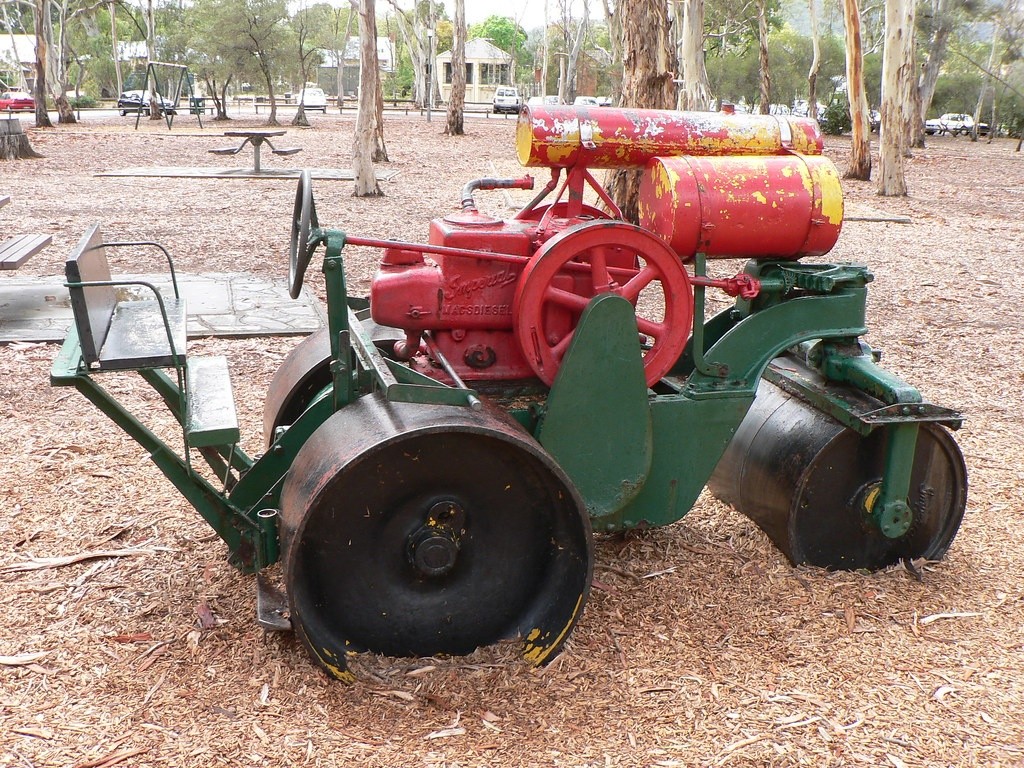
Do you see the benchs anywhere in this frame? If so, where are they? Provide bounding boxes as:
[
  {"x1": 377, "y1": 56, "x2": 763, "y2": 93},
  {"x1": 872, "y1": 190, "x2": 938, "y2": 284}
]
[
  {"x1": 0, "y1": 233, "x2": 53, "y2": 271},
  {"x1": 232, "y1": 95, "x2": 519, "y2": 121},
  {"x1": 61, "y1": 225, "x2": 190, "y2": 370},
  {"x1": 208, "y1": 146, "x2": 238, "y2": 155},
  {"x1": 271, "y1": 148, "x2": 302, "y2": 155}
]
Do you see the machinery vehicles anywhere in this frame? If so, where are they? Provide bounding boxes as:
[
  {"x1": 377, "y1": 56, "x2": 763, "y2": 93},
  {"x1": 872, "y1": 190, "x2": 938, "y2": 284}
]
[{"x1": 48, "y1": 102, "x2": 968, "y2": 690}]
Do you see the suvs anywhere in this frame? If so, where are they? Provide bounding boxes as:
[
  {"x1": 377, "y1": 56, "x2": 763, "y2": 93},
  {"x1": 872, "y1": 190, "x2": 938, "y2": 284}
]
[
  {"x1": 925, "y1": 113, "x2": 975, "y2": 136},
  {"x1": 493, "y1": 86, "x2": 520, "y2": 112}
]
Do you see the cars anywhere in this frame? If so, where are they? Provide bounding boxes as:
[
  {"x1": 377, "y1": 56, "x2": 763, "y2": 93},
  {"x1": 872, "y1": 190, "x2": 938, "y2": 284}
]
[
  {"x1": 974, "y1": 122, "x2": 989, "y2": 136},
  {"x1": 549, "y1": 95, "x2": 613, "y2": 108},
  {"x1": 0, "y1": 92, "x2": 36, "y2": 113},
  {"x1": 709, "y1": 98, "x2": 881, "y2": 124},
  {"x1": 297, "y1": 88, "x2": 327, "y2": 114},
  {"x1": 118, "y1": 89, "x2": 176, "y2": 117}
]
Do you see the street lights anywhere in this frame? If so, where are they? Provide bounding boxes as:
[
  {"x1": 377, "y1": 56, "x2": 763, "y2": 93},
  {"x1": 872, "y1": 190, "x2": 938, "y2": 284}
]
[{"x1": 427, "y1": 26, "x2": 433, "y2": 123}]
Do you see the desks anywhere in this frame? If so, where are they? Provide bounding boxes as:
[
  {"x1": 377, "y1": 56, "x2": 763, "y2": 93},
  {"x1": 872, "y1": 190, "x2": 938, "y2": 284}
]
[{"x1": 223, "y1": 129, "x2": 288, "y2": 173}]
